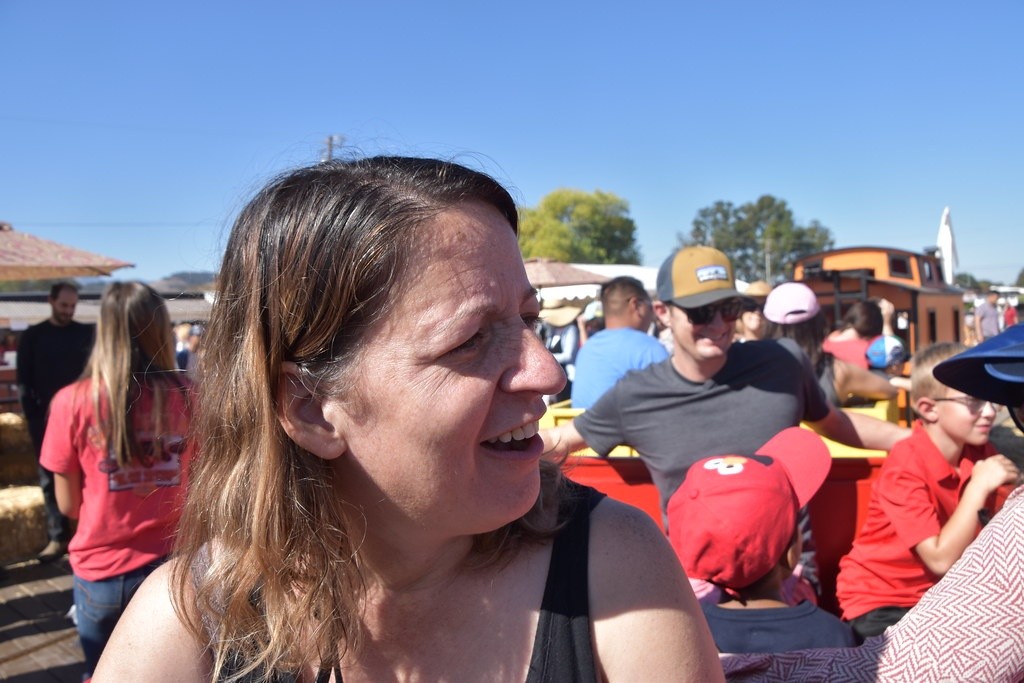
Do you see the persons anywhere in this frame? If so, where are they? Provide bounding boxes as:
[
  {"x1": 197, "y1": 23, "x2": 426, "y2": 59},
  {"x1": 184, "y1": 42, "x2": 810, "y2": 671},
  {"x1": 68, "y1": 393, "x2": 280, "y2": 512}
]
[
  {"x1": 536, "y1": 245, "x2": 1024, "y2": 683},
  {"x1": 17, "y1": 276, "x2": 204, "y2": 682},
  {"x1": 91, "y1": 157, "x2": 728, "y2": 683}
]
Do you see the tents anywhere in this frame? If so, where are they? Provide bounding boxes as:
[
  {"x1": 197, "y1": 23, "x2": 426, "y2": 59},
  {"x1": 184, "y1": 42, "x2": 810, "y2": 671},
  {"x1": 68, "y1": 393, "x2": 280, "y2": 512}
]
[{"x1": 523, "y1": 262, "x2": 750, "y2": 301}]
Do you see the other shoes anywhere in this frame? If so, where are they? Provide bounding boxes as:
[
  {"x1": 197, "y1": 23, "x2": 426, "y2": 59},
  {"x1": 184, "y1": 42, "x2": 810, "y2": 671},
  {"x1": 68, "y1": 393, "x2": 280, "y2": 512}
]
[{"x1": 37, "y1": 539, "x2": 68, "y2": 562}]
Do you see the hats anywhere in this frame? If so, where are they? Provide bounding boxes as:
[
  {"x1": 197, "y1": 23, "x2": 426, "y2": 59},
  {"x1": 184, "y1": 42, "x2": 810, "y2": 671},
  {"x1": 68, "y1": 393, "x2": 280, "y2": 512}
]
[
  {"x1": 865, "y1": 336, "x2": 912, "y2": 369},
  {"x1": 659, "y1": 245, "x2": 745, "y2": 309},
  {"x1": 763, "y1": 282, "x2": 819, "y2": 325},
  {"x1": 582, "y1": 301, "x2": 606, "y2": 321},
  {"x1": 664, "y1": 426, "x2": 832, "y2": 588},
  {"x1": 536, "y1": 296, "x2": 582, "y2": 325},
  {"x1": 932, "y1": 323, "x2": 1024, "y2": 404}
]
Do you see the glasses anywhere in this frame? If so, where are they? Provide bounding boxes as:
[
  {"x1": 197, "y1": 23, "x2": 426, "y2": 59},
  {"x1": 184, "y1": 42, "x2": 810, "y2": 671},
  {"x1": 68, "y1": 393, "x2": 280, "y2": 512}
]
[
  {"x1": 932, "y1": 392, "x2": 1003, "y2": 416},
  {"x1": 672, "y1": 297, "x2": 740, "y2": 327}
]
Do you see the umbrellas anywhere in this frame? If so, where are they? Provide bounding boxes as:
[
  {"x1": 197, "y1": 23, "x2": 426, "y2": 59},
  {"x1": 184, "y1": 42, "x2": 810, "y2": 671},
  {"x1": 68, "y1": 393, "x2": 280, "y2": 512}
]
[{"x1": 0, "y1": 222, "x2": 135, "y2": 281}]
